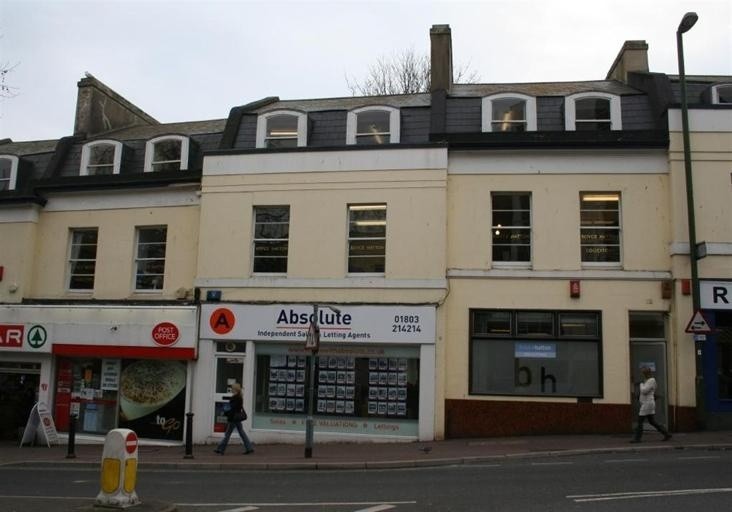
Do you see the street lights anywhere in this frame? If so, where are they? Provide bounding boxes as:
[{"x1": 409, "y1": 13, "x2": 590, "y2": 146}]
[{"x1": 675, "y1": 11, "x2": 710, "y2": 436}]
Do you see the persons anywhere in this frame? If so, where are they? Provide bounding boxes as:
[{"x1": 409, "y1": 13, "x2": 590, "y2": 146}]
[
  {"x1": 628, "y1": 367, "x2": 672, "y2": 443},
  {"x1": 212, "y1": 382, "x2": 256, "y2": 457}
]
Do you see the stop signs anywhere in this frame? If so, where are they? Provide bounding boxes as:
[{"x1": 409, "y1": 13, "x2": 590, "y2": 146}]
[{"x1": 124, "y1": 430, "x2": 140, "y2": 455}]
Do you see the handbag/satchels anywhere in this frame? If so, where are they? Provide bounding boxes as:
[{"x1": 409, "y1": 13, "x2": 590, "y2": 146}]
[{"x1": 233, "y1": 412, "x2": 246, "y2": 423}]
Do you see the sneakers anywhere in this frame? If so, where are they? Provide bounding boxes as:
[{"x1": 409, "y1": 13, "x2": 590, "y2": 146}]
[
  {"x1": 630, "y1": 440, "x2": 642, "y2": 443},
  {"x1": 662, "y1": 434, "x2": 672, "y2": 441},
  {"x1": 213, "y1": 450, "x2": 224, "y2": 455},
  {"x1": 242, "y1": 450, "x2": 254, "y2": 455}
]
